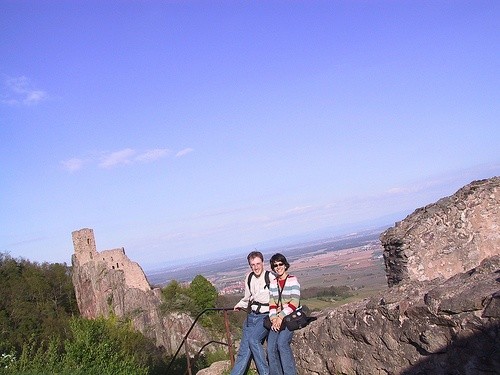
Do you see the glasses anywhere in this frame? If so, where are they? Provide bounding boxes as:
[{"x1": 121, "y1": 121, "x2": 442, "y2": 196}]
[{"x1": 273, "y1": 263, "x2": 285, "y2": 267}]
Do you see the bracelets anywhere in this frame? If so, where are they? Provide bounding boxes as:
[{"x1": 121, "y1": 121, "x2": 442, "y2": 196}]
[{"x1": 277, "y1": 315, "x2": 284, "y2": 321}]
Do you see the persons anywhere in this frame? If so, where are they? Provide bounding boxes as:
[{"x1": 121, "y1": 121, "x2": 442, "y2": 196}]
[
  {"x1": 267, "y1": 252, "x2": 303, "y2": 375},
  {"x1": 228, "y1": 252, "x2": 278, "y2": 375}
]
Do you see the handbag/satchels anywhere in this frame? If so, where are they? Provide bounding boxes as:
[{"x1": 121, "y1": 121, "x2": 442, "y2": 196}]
[{"x1": 282, "y1": 309, "x2": 310, "y2": 331}]
